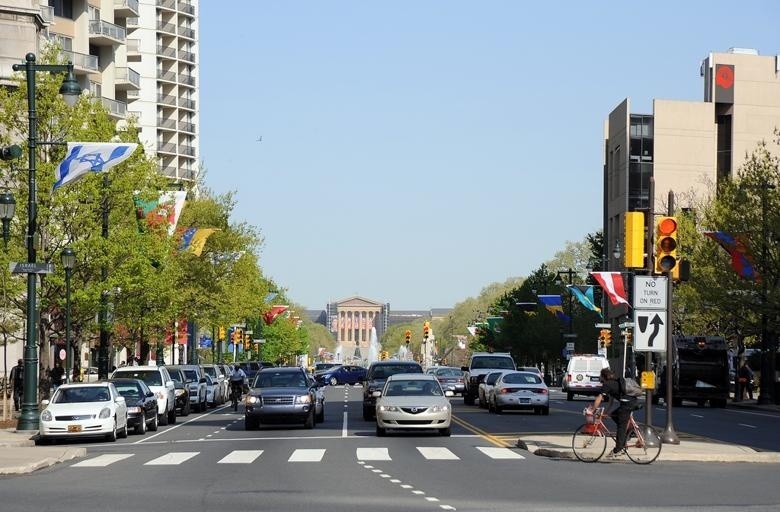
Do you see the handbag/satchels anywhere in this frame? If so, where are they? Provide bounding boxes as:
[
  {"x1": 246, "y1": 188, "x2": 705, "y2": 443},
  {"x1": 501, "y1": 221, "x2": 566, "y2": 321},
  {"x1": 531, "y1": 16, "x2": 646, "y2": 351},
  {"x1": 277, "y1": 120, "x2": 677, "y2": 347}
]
[
  {"x1": 619, "y1": 376, "x2": 643, "y2": 397},
  {"x1": 739, "y1": 378, "x2": 747, "y2": 383}
]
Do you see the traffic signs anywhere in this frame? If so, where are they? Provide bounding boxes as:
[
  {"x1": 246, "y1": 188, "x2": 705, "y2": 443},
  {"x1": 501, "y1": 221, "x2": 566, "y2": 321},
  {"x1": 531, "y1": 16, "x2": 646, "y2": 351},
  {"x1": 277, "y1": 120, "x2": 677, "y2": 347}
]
[
  {"x1": 618, "y1": 320, "x2": 635, "y2": 343},
  {"x1": 595, "y1": 323, "x2": 611, "y2": 328},
  {"x1": 633, "y1": 309, "x2": 667, "y2": 354},
  {"x1": 634, "y1": 274, "x2": 668, "y2": 311}
]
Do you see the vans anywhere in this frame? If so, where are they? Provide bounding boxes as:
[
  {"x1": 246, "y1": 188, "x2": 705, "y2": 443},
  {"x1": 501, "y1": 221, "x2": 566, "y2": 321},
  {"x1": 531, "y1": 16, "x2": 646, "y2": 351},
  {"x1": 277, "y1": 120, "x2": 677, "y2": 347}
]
[{"x1": 565, "y1": 354, "x2": 613, "y2": 401}]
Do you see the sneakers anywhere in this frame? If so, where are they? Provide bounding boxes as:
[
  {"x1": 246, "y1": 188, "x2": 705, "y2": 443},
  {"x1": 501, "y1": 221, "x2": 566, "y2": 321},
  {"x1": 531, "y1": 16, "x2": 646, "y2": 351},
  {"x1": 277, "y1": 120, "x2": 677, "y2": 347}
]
[{"x1": 606, "y1": 448, "x2": 625, "y2": 457}]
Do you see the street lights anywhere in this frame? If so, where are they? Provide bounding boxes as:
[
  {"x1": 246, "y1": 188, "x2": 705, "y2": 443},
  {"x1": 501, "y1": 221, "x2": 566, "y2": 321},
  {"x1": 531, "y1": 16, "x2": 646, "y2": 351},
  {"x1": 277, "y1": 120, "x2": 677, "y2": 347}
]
[
  {"x1": 552, "y1": 268, "x2": 577, "y2": 356},
  {"x1": 732, "y1": 182, "x2": 776, "y2": 407},
  {"x1": 532, "y1": 273, "x2": 549, "y2": 380},
  {"x1": 467, "y1": 295, "x2": 519, "y2": 330},
  {"x1": 0, "y1": 193, "x2": 15, "y2": 251},
  {"x1": 12, "y1": 51, "x2": 84, "y2": 435},
  {"x1": 59, "y1": 247, "x2": 77, "y2": 384},
  {"x1": 611, "y1": 239, "x2": 625, "y2": 258}
]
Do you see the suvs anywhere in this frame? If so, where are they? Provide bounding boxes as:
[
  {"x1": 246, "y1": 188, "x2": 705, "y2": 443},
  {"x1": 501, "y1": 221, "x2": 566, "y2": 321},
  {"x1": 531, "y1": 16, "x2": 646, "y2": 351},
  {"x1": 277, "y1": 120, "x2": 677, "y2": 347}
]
[
  {"x1": 113, "y1": 368, "x2": 176, "y2": 429},
  {"x1": 244, "y1": 365, "x2": 318, "y2": 430}
]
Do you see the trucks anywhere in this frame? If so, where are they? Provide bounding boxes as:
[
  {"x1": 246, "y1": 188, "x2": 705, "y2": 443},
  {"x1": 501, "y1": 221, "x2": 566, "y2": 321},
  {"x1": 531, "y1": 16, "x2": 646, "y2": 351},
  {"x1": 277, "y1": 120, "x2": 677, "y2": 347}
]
[
  {"x1": 652, "y1": 335, "x2": 730, "y2": 409},
  {"x1": 729, "y1": 348, "x2": 761, "y2": 381}
]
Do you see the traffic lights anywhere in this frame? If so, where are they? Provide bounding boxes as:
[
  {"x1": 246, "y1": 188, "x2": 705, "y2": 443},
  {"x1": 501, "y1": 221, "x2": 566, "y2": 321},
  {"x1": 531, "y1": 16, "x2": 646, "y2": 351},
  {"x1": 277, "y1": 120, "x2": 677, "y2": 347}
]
[
  {"x1": 653, "y1": 215, "x2": 681, "y2": 280},
  {"x1": 245, "y1": 335, "x2": 251, "y2": 350},
  {"x1": 405, "y1": 330, "x2": 411, "y2": 344},
  {"x1": 600, "y1": 335, "x2": 606, "y2": 349},
  {"x1": 382, "y1": 351, "x2": 386, "y2": 359},
  {"x1": 231, "y1": 332, "x2": 235, "y2": 343},
  {"x1": 236, "y1": 328, "x2": 244, "y2": 344},
  {"x1": 605, "y1": 330, "x2": 613, "y2": 347},
  {"x1": 254, "y1": 344, "x2": 258, "y2": 352},
  {"x1": 219, "y1": 326, "x2": 227, "y2": 342},
  {"x1": 424, "y1": 321, "x2": 431, "y2": 338}
]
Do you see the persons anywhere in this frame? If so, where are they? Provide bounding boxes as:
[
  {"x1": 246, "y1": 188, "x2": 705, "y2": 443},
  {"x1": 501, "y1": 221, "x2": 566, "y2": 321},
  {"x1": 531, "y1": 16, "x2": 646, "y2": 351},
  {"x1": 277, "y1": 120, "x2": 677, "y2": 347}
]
[
  {"x1": 590, "y1": 368, "x2": 638, "y2": 457},
  {"x1": 9, "y1": 359, "x2": 25, "y2": 411},
  {"x1": 39, "y1": 359, "x2": 85, "y2": 403},
  {"x1": 229, "y1": 362, "x2": 246, "y2": 408},
  {"x1": 738, "y1": 362, "x2": 755, "y2": 401},
  {"x1": 112, "y1": 355, "x2": 140, "y2": 372}
]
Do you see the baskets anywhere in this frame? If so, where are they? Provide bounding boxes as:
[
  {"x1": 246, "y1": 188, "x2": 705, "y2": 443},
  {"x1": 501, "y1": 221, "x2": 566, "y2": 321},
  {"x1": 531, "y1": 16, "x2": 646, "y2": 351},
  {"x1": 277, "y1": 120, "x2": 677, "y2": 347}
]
[{"x1": 586, "y1": 412, "x2": 594, "y2": 424}]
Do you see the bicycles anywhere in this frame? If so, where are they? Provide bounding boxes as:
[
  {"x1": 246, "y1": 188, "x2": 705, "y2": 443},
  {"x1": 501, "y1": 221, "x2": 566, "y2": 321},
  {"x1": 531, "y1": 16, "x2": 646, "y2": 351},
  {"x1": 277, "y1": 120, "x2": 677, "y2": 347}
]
[{"x1": 572, "y1": 399, "x2": 662, "y2": 466}]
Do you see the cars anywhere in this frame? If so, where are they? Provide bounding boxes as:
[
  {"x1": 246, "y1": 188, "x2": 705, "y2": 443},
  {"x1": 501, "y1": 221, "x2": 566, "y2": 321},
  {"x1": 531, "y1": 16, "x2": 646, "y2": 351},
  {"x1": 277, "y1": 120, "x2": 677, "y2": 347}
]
[
  {"x1": 370, "y1": 373, "x2": 454, "y2": 438},
  {"x1": 60, "y1": 366, "x2": 113, "y2": 384},
  {"x1": 104, "y1": 376, "x2": 160, "y2": 436},
  {"x1": 36, "y1": 382, "x2": 129, "y2": 441},
  {"x1": 562, "y1": 372, "x2": 567, "y2": 392}
]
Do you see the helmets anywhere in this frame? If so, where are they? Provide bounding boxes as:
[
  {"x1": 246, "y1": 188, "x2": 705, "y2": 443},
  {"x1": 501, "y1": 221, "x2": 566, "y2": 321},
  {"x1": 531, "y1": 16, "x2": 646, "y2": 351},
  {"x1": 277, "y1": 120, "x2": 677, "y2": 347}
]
[{"x1": 234, "y1": 362, "x2": 240, "y2": 367}]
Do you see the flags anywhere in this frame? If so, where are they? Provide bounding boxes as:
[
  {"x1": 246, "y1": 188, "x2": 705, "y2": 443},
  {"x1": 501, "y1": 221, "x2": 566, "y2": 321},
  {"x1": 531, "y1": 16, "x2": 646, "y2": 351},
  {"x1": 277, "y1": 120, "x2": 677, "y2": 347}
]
[
  {"x1": 536, "y1": 295, "x2": 572, "y2": 320},
  {"x1": 702, "y1": 231, "x2": 762, "y2": 284},
  {"x1": 170, "y1": 226, "x2": 215, "y2": 258},
  {"x1": 588, "y1": 271, "x2": 633, "y2": 309},
  {"x1": 132, "y1": 190, "x2": 187, "y2": 260},
  {"x1": 451, "y1": 301, "x2": 539, "y2": 350},
  {"x1": 261, "y1": 293, "x2": 302, "y2": 332},
  {"x1": 566, "y1": 285, "x2": 604, "y2": 319},
  {"x1": 50, "y1": 141, "x2": 139, "y2": 194},
  {"x1": 207, "y1": 251, "x2": 245, "y2": 280}
]
